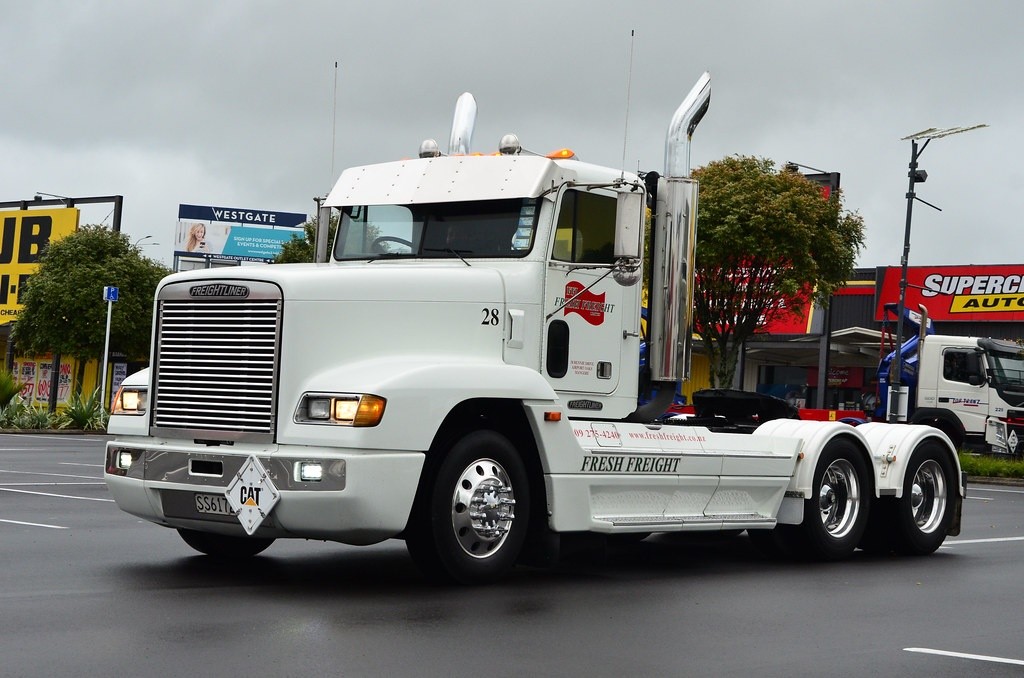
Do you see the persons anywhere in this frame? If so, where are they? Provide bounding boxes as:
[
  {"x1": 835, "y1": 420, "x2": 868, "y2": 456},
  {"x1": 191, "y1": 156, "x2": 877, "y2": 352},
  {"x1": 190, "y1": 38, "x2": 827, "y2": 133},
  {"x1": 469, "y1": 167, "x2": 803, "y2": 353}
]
[
  {"x1": 945, "y1": 353, "x2": 969, "y2": 381},
  {"x1": 430, "y1": 224, "x2": 465, "y2": 256},
  {"x1": 185, "y1": 222, "x2": 209, "y2": 253}
]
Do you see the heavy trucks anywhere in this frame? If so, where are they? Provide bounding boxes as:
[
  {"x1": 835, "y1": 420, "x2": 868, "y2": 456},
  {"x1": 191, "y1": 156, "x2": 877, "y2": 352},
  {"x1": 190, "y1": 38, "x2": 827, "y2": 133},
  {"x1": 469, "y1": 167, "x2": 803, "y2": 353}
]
[{"x1": 103, "y1": 62, "x2": 969, "y2": 587}]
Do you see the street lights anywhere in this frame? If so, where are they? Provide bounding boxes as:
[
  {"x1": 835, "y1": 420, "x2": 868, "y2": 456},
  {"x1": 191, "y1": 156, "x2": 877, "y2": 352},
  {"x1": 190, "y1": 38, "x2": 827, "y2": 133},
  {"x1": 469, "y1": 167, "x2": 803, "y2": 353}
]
[{"x1": 884, "y1": 121, "x2": 991, "y2": 423}]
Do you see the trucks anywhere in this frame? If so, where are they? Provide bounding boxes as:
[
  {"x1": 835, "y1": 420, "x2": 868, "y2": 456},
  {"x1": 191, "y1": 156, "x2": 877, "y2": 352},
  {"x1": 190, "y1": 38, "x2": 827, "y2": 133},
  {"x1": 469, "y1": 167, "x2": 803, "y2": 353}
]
[{"x1": 837, "y1": 301, "x2": 1024, "y2": 460}]
